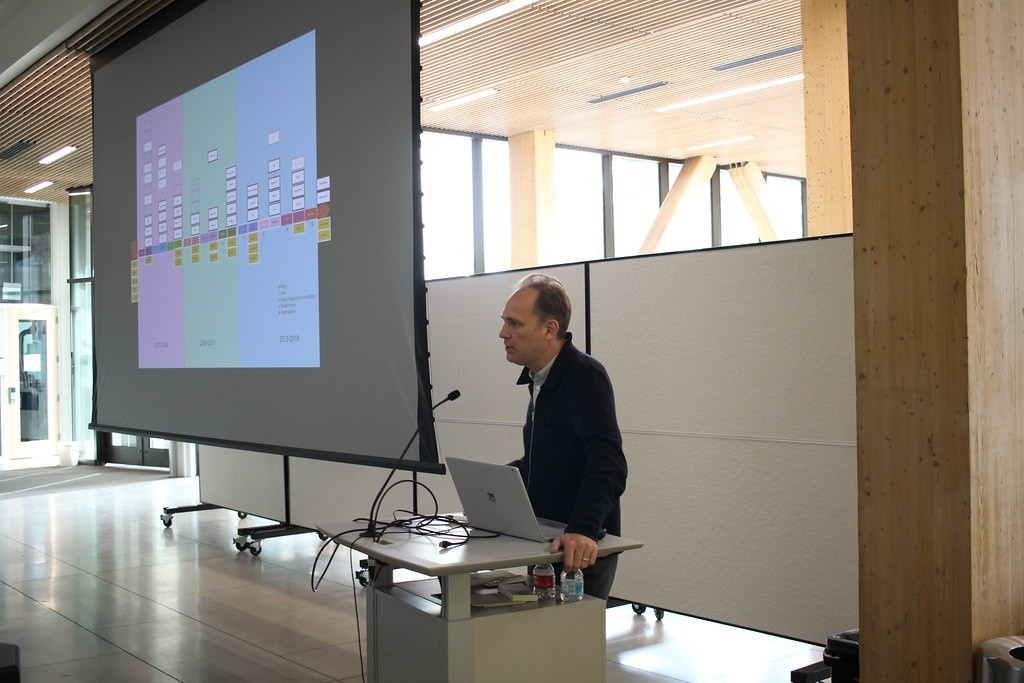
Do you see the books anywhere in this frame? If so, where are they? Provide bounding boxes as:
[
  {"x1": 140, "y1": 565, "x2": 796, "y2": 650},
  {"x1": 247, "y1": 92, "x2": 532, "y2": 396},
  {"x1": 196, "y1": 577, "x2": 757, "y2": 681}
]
[{"x1": 499, "y1": 583, "x2": 538, "y2": 601}]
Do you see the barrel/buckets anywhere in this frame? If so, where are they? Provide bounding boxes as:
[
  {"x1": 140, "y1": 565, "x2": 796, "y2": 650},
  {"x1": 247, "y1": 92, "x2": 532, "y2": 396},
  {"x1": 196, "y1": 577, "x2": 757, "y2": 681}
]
[{"x1": 58, "y1": 441, "x2": 80, "y2": 467}]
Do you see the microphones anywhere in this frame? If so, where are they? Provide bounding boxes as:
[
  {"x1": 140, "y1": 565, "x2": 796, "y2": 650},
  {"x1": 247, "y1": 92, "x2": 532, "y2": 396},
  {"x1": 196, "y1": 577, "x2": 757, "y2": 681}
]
[{"x1": 361, "y1": 390, "x2": 461, "y2": 538}]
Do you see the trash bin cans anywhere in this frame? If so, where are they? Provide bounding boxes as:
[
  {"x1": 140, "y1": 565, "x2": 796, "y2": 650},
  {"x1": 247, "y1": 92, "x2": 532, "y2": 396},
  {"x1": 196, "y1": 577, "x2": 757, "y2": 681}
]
[{"x1": 58, "y1": 440, "x2": 80, "y2": 466}]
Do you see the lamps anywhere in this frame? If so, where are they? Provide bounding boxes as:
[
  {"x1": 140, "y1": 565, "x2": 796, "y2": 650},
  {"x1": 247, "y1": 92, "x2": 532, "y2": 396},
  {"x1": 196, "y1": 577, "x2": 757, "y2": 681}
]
[
  {"x1": 428, "y1": 87, "x2": 498, "y2": 112},
  {"x1": 24, "y1": 181, "x2": 54, "y2": 194},
  {"x1": 38, "y1": 144, "x2": 79, "y2": 164}
]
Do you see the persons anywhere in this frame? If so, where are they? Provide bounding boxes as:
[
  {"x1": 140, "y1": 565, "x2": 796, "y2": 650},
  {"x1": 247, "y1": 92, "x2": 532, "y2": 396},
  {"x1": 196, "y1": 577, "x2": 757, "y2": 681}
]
[{"x1": 499, "y1": 273, "x2": 628, "y2": 602}]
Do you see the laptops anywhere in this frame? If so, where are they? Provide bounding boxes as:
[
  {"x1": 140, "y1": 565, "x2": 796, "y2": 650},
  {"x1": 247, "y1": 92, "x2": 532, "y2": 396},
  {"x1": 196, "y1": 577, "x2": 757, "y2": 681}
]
[{"x1": 446, "y1": 456, "x2": 568, "y2": 543}]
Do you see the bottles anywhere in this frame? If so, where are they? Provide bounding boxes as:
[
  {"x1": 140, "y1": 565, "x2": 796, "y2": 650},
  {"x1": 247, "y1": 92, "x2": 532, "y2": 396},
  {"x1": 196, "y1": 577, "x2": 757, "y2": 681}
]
[
  {"x1": 533, "y1": 563, "x2": 556, "y2": 600},
  {"x1": 560, "y1": 563, "x2": 583, "y2": 602}
]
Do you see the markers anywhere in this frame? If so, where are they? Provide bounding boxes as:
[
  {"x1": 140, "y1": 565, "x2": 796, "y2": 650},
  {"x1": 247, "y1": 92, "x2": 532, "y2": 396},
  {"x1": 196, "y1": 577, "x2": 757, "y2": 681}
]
[{"x1": 446, "y1": 514, "x2": 468, "y2": 521}]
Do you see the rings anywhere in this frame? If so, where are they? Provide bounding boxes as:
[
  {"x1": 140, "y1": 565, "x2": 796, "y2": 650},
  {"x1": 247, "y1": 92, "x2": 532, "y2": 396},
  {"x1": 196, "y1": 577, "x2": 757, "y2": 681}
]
[{"x1": 582, "y1": 558, "x2": 589, "y2": 561}]
